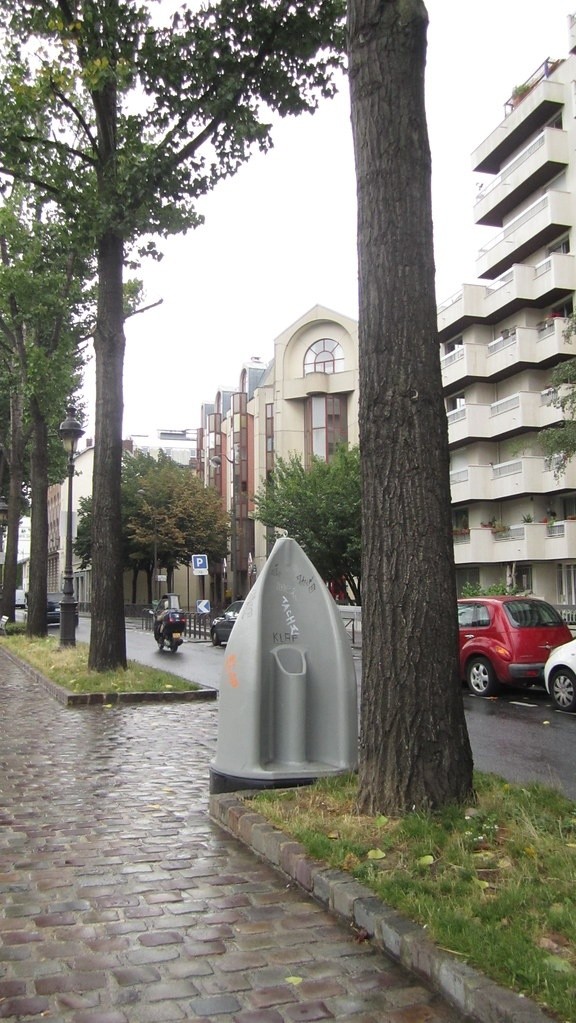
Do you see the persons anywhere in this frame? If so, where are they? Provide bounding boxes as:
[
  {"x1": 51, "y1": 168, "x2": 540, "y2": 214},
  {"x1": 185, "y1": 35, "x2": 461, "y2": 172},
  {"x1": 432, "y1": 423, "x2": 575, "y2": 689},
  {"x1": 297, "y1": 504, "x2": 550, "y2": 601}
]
[{"x1": 156, "y1": 594, "x2": 169, "y2": 644}]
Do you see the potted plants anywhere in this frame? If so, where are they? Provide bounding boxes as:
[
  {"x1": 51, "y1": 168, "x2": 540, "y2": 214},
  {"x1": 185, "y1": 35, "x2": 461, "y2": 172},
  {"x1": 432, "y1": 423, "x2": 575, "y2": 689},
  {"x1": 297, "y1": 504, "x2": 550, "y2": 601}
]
[
  {"x1": 499, "y1": 325, "x2": 517, "y2": 339},
  {"x1": 480, "y1": 517, "x2": 510, "y2": 535},
  {"x1": 536, "y1": 312, "x2": 560, "y2": 331}
]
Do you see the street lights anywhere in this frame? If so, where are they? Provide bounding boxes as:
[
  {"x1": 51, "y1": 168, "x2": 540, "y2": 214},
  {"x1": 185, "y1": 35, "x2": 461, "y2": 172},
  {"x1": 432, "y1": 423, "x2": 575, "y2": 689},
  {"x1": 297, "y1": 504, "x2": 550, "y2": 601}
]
[
  {"x1": 137, "y1": 490, "x2": 157, "y2": 607},
  {"x1": 210, "y1": 452, "x2": 236, "y2": 602},
  {"x1": 57, "y1": 404, "x2": 86, "y2": 645}
]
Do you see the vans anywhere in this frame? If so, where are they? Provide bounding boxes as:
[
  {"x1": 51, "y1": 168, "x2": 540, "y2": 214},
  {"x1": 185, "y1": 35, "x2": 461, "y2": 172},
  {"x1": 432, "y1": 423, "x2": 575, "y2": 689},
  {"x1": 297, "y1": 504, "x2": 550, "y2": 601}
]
[{"x1": 15, "y1": 590, "x2": 26, "y2": 608}]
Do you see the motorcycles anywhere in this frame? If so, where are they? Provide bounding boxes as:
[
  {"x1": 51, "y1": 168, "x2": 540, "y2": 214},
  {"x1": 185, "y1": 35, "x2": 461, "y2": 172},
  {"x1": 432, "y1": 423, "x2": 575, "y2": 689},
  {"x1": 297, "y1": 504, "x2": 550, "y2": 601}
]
[{"x1": 147, "y1": 593, "x2": 187, "y2": 652}]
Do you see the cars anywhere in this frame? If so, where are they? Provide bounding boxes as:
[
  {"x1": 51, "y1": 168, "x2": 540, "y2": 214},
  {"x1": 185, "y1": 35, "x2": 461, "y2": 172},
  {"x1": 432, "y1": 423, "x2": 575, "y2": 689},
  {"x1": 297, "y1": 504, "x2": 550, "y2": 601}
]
[
  {"x1": 457, "y1": 596, "x2": 574, "y2": 697},
  {"x1": 210, "y1": 600, "x2": 245, "y2": 645},
  {"x1": 544, "y1": 636, "x2": 575, "y2": 710},
  {"x1": 23, "y1": 592, "x2": 79, "y2": 627}
]
